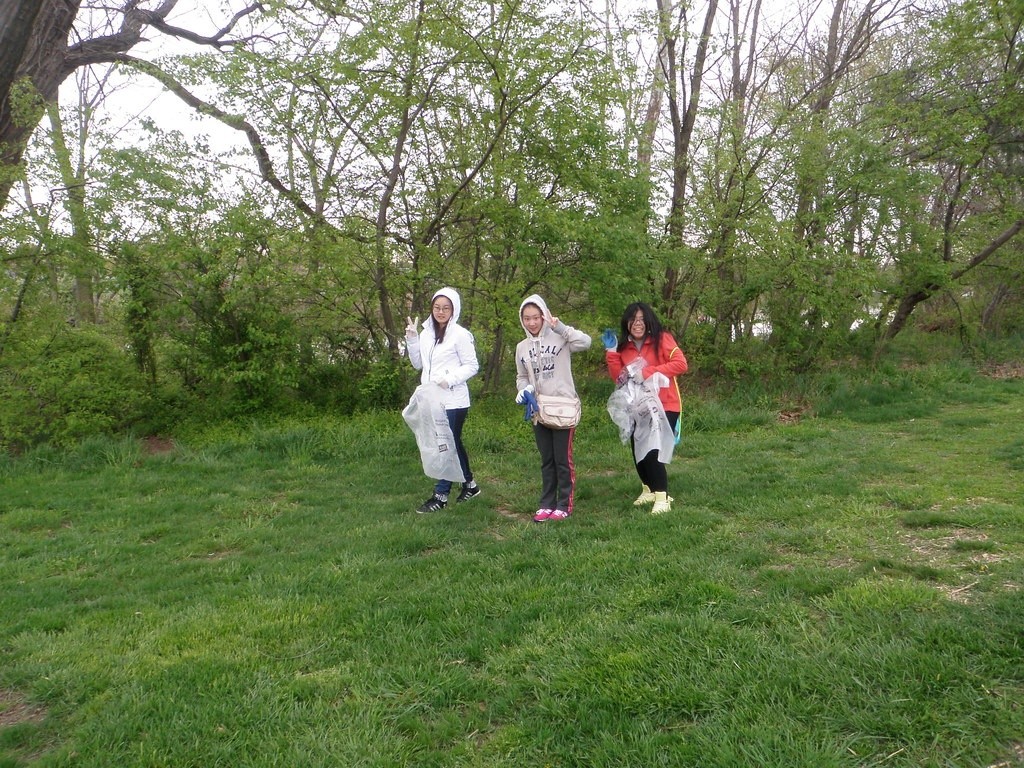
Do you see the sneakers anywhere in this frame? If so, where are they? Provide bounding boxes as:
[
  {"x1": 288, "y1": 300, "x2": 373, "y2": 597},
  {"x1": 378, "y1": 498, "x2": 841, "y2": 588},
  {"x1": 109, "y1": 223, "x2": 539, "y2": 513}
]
[
  {"x1": 533, "y1": 507, "x2": 555, "y2": 522},
  {"x1": 549, "y1": 508, "x2": 571, "y2": 521},
  {"x1": 416, "y1": 491, "x2": 448, "y2": 515},
  {"x1": 651, "y1": 490, "x2": 675, "y2": 516},
  {"x1": 455, "y1": 483, "x2": 482, "y2": 505},
  {"x1": 632, "y1": 482, "x2": 656, "y2": 506}
]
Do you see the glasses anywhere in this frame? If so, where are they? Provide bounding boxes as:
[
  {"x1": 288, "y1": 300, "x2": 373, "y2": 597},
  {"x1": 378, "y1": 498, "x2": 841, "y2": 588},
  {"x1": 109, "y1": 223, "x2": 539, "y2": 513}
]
[{"x1": 432, "y1": 305, "x2": 453, "y2": 314}]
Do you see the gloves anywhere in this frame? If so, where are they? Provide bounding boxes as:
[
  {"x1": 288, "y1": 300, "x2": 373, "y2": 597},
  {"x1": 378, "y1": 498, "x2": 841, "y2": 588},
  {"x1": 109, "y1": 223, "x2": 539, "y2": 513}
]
[
  {"x1": 405, "y1": 316, "x2": 419, "y2": 339},
  {"x1": 435, "y1": 376, "x2": 449, "y2": 389},
  {"x1": 601, "y1": 328, "x2": 618, "y2": 352},
  {"x1": 632, "y1": 368, "x2": 645, "y2": 385},
  {"x1": 514, "y1": 384, "x2": 539, "y2": 422}
]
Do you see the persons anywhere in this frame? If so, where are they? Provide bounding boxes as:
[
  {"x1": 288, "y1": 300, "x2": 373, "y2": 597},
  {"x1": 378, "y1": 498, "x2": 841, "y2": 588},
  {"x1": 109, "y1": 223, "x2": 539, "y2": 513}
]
[
  {"x1": 405, "y1": 287, "x2": 481, "y2": 514},
  {"x1": 601, "y1": 303, "x2": 688, "y2": 515},
  {"x1": 515, "y1": 293, "x2": 591, "y2": 522}
]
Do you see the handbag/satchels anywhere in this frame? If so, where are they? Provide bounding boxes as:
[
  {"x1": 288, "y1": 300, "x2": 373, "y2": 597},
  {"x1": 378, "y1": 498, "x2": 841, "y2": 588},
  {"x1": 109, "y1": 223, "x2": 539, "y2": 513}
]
[
  {"x1": 402, "y1": 381, "x2": 467, "y2": 484},
  {"x1": 606, "y1": 372, "x2": 676, "y2": 466},
  {"x1": 531, "y1": 392, "x2": 582, "y2": 429}
]
